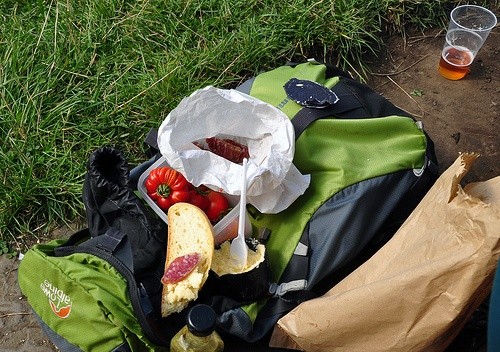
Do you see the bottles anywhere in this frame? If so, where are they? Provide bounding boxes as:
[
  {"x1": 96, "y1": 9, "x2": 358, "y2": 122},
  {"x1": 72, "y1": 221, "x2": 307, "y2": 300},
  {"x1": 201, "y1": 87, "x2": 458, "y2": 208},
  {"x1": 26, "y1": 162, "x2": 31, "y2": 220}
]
[{"x1": 169, "y1": 304, "x2": 224, "y2": 352}]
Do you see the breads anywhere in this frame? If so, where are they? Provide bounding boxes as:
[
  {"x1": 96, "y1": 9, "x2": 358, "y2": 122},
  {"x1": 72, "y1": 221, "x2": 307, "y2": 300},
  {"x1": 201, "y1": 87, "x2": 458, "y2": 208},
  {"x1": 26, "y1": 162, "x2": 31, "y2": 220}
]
[{"x1": 161, "y1": 202, "x2": 215, "y2": 317}]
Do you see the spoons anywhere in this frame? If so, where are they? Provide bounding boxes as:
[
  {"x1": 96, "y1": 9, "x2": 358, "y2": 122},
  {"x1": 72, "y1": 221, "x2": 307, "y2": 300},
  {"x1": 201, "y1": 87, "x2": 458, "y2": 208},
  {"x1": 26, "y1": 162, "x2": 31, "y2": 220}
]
[{"x1": 229, "y1": 158, "x2": 249, "y2": 272}]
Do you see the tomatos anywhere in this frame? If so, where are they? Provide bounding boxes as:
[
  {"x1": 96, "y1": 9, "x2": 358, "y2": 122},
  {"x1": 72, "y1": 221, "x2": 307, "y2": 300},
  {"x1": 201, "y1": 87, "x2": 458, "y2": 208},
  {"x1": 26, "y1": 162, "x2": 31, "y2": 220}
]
[{"x1": 145, "y1": 166, "x2": 233, "y2": 223}]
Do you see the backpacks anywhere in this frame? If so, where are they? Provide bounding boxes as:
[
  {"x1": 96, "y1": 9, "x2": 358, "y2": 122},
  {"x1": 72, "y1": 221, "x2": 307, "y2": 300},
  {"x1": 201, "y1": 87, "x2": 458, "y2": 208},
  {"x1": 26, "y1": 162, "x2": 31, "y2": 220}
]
[{"x1": 16, "y1": 57, "x2": 439, "y2": 351}]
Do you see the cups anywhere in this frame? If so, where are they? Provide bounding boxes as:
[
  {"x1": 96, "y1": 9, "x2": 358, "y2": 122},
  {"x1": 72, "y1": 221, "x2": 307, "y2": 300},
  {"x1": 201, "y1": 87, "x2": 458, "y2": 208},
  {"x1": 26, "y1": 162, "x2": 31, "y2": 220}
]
[{"x1": 438, "y1": 4, "x2": 498, "y2": 81}]
